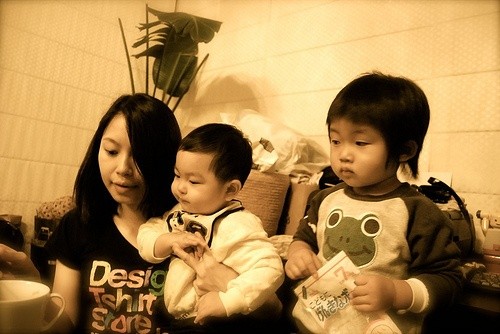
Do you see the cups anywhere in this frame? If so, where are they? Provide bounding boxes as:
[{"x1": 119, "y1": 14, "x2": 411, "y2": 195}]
[{"x1": 0, "y1": 280, "x2": 65, "y2": 334}]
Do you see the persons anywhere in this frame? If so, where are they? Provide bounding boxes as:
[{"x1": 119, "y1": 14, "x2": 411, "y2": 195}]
[
  {"x1": 137, "y1": 123, "x2": 283, "y2": 334},
  {"x1": 0, "y1": 92, "x2": 284, "y2": 334},
  {"x1": 285, "y1": 71, "x2": 466, "y2": 334}
]
